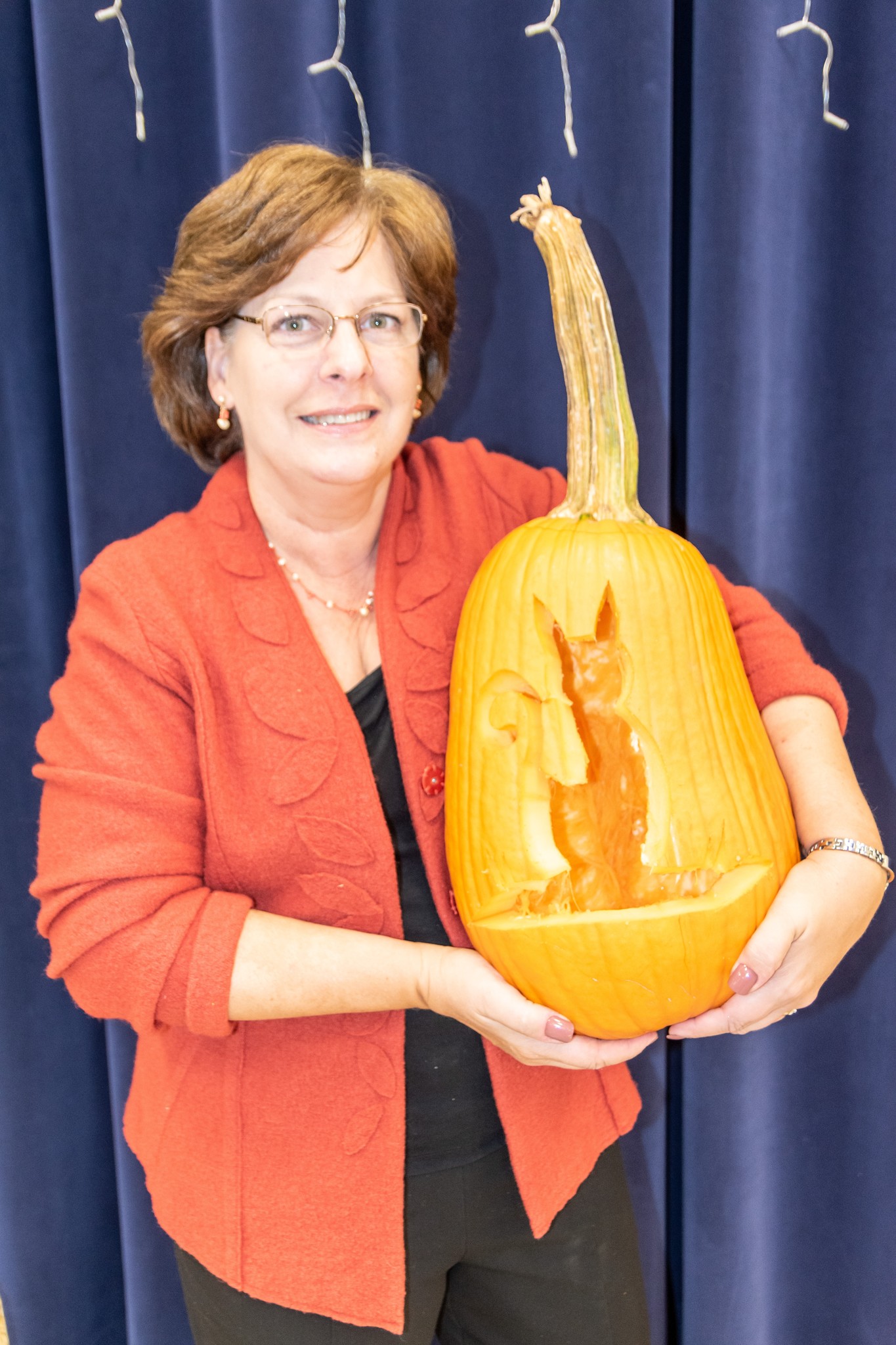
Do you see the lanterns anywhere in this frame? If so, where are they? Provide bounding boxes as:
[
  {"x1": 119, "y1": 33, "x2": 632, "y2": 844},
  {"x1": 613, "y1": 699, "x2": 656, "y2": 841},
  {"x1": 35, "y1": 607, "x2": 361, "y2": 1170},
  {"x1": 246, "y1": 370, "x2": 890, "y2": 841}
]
[{"x1": 443, "y1": 169, "x2": 806, "y2": 1040}]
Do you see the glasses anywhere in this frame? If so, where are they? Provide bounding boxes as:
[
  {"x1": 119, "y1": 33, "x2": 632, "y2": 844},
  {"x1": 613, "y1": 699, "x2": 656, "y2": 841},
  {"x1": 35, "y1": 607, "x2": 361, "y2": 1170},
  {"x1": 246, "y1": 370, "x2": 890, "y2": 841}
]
[{"x1": 230, "y1": 301, "x2": 430, "y2": 351}]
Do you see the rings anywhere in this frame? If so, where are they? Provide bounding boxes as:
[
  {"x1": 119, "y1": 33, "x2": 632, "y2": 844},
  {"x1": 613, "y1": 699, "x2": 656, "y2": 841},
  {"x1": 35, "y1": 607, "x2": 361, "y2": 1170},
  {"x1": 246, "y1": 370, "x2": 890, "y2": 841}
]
[{"x1": 784, "y1": 1008, "x2": 798, "y2": 1016}]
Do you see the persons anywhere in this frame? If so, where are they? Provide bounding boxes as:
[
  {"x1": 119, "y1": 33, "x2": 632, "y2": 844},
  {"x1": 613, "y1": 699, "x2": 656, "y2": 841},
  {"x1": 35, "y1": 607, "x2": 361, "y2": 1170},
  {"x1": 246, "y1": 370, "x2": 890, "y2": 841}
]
[{"x1": 31, "y1": 143, "x2": 896, "y2": 1345}]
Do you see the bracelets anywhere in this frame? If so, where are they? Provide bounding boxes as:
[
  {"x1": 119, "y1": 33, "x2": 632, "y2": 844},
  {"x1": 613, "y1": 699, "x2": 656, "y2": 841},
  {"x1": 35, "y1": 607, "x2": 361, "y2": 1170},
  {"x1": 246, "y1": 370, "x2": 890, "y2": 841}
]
[{"x1": 805, "y1": 837, "x2": 896, "y2": 882}]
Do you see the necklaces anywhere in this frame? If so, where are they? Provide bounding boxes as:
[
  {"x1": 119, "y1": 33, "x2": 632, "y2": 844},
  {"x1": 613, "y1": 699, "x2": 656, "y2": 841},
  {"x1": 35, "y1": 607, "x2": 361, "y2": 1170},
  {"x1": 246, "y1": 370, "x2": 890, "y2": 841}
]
[{"x1": 267, "y1": 540, "x2": 378, "y2": 681}]
